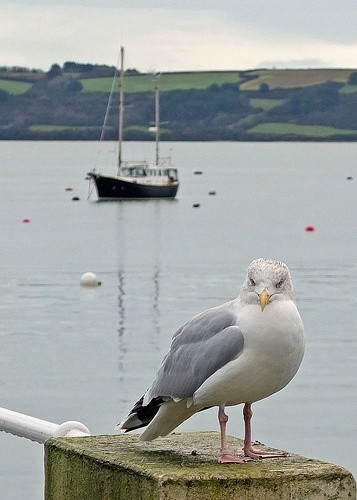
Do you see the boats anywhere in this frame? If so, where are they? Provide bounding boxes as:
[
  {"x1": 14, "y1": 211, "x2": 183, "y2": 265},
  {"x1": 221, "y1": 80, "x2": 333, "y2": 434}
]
[{"x1": 88, "y1": 45, "x2": 178, "y2": 200}]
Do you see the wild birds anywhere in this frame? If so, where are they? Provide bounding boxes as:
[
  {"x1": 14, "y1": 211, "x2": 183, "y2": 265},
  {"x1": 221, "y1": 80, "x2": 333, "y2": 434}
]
[{"x1": 114, "y1": 257, "x2": 305, "y2": 465}]
